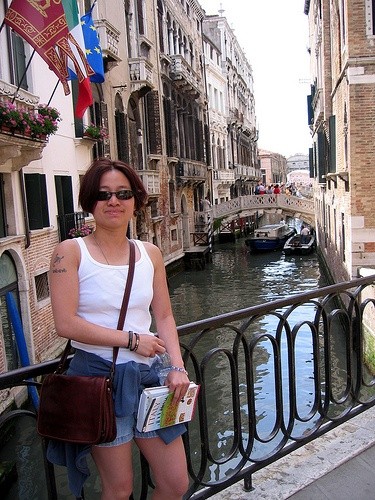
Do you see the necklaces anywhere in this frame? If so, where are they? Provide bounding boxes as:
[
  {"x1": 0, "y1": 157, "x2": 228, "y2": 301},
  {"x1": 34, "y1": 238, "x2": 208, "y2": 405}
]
[{"x1": 91, "y1": 232, "x2": 110, "y2": 266}]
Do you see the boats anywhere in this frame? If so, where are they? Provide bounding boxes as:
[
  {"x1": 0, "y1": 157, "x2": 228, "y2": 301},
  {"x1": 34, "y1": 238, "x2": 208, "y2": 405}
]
[
  {"x1": 244, "y1": 223, "x2": 297, "y2": 253},
  {"x1": 282, "y1": 225, "x2": 316, "y2": 255}
]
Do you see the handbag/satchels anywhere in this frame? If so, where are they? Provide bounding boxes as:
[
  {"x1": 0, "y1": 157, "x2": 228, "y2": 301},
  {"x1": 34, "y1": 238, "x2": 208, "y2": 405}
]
[{"x1": 36, "y1": 374, "x2": 121, "y2": 444}]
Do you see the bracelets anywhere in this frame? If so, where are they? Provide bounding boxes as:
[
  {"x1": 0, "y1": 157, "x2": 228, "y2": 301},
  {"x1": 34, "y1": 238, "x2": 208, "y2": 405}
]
[
  {"x1": 128, "y1": 331, "x2": 133, "y2": 349},
  {"x1": 130, "y1": 333, "x2": 140, "y2": 352}
]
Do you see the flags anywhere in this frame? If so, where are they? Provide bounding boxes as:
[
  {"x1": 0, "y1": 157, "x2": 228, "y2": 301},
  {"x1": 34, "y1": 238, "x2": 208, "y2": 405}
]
[
  {"x1": 4, "y1": 0, "x2": 96, "y2": 120},
  {"x1": 50, "y1": 12, "x2": 105, "y2": 84}
]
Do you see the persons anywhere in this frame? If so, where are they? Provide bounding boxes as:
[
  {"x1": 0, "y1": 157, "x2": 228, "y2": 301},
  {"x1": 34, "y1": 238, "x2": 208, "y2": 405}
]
[
  {"x1": 50, "y1": 161, "x2": 200, "y2": 500},
  {"x1": 301, "y1": 226, "x2": 310, "y2": 243},
  {"x1": 279, "y1": 218, "x2": 285, "y2": 226},
  {"x1": 255, "y1": 182, "x2": 297, "y2": 197}
]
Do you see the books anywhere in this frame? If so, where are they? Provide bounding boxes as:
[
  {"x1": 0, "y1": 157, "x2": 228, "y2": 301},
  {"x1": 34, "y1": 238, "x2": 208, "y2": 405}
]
[{"x1": 136, "y1": 381, "x2": 200, "y2": 433}]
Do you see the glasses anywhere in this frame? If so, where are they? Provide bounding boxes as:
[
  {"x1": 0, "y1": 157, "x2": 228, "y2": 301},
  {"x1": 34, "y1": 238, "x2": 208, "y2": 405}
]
[{"x1": 98, "y1": 190, "x2": 134, "y2": 201}]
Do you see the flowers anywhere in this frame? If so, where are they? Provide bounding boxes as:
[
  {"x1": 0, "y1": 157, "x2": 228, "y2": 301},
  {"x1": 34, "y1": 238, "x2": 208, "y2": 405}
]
[
  {"x1": 83, "y1": 123, "x2": 110, "y2": 141},
  {"x1": 68, "y1": 224, "x2": 94, "y2": 237},
  {"x1": 0, "y1": 99, "x2": 63, "y2": 141}
]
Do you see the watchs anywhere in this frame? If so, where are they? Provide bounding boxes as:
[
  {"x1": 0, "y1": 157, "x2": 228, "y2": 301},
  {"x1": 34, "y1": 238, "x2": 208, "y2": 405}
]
[{"x1": 169, "y1": 366, "x2": 189, "y2": 375}]
